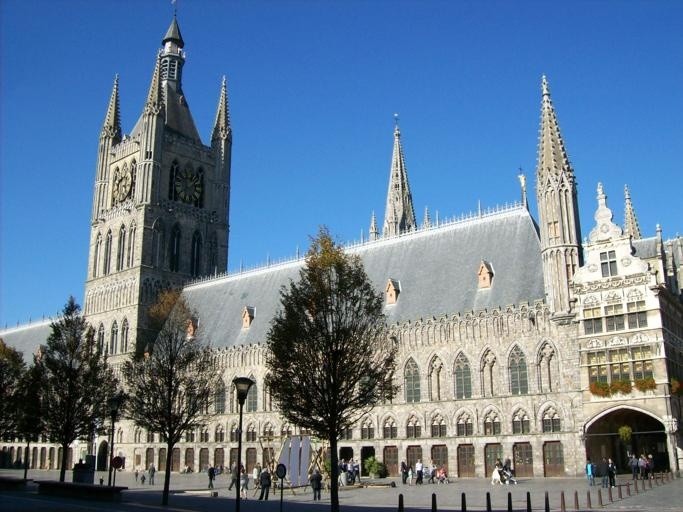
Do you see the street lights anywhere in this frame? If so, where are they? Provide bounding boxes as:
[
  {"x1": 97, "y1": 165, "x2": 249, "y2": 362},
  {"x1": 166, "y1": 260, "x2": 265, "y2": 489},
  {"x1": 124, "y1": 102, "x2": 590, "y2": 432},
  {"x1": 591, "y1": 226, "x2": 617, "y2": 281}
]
[
  {"x1": 233, "y1": 376, "x2": 255, "y2": 510},
  {"x1": 107, "y1": 395, "x2": 122, "y2": 486}
]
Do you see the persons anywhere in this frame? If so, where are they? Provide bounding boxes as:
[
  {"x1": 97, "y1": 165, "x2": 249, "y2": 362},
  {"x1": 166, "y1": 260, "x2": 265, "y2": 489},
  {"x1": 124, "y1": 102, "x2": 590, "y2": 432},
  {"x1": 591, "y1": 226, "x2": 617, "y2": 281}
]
[
  {"x1": 399, "y1": 457, "x2": 450, "y2": 486},
  {"x1": 133, "y1": 463, "x2": 156, "y2": 485},
  {"x1": 308, "y1": 467, "x2": 322, "y2": 500},
  {"x1": 79, "y1": 458, "x2": 82, "y2": 464},
  {"x1": 201, "y1": 461, "x2": 279, "y2": 501},
  {"x1": 585, "y1": 456, "x2": 617, "y2": 489},
  {"x1": 179, "y1": 464, "x2": 194, "y2": 475},
  {"x1": 490, "y1": 458, "x2": 518, "y2": 485},
  {"x1": 627, "y1": 453, "x2": 655, "y2": 481},
  {"x1": 338, "y1": 457, "x2": 363, "y2": 486},
  {"x1": 99, "y1": 476, "x2": 104, "y2": 484},
  {"x1": 45, "y1": 456, "x2": 52, "y2": 470}
]
